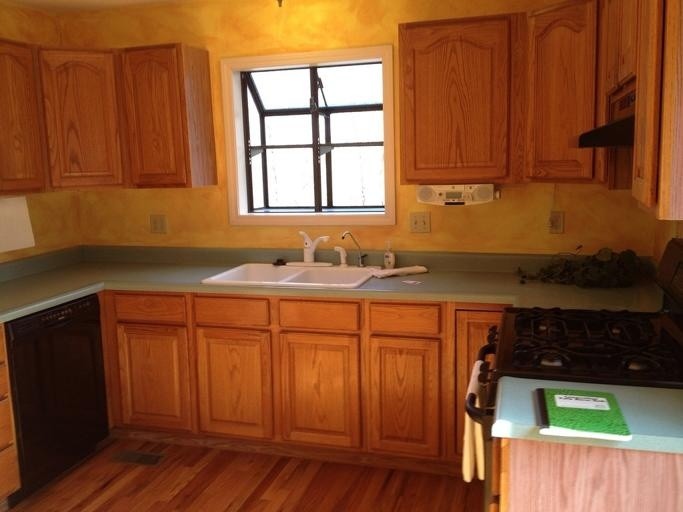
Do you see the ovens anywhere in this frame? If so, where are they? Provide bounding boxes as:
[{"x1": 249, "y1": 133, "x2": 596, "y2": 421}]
[{"x1": 2, "y1": 295, "x2": 112, "y2": 493}]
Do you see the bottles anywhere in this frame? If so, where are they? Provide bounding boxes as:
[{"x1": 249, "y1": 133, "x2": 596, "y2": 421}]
[{"x1": 383, "y1": 247, "x2": 395, "y2": 269}]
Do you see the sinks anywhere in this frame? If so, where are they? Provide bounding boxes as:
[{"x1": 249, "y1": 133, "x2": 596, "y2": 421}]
[
  {"x1": 200, "y1": 262, "x2": 304, "y2": 287},
  {"x1": 279, "y1": 264, "x2": 382, "y2": 289}
]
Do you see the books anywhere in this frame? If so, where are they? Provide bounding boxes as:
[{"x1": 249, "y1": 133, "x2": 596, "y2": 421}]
[{"x1": 535, "y1": 388, "x2": 630, "y2": 441}]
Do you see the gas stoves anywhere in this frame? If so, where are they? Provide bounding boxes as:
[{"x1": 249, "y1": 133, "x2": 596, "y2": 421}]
[{"x1": 479, "y1": 301, "x2": 677, "y2": 393}]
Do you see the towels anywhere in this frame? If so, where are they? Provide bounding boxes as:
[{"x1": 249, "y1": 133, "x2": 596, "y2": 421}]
[{"x1": 461, "y1": 360, "x2": 484, "y2": 485}]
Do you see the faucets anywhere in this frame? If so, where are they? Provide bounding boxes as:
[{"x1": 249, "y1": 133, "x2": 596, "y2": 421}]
[
  {"x1": 334, "y1": 247, "x2": 348, "y2": 267},
  {"x1": 341, "y1": 231, "x2": 368, "y2": 266},
  {"x1": 298, "y1": 230, "x2": 330, "y2": 262}
]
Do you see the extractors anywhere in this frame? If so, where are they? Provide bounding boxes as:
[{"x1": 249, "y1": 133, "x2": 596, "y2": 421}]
[{"x1": 577, "y1": 113, "x2": 635, "y2": 150}]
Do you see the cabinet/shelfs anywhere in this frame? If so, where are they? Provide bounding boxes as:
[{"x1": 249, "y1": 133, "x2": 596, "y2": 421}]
[
  {"x1": 446, "y1": 303, "x2": 507, "y2": 476},
  {"x1": 0, "y1": 323, "x2": 22, "y2": 501},
  {"x1": 626, "y1": 0, "x2": 682, "y2": 220},
  {"x1": 361, "y1": 300, "x2": 447, "y2": 475},
  {"x1": 272, "y1": 296, "x2": 363, "y2": 462},
  {"x1": 0, "y1": 35, "x2": 46, "y2": 198},
  {"x1": 597, "y1": 0, "x2": 638, "y2": 95},
  {"x1": 117, "y1": 41, "x2": 217, "y2": 189},
  {"x1": 35, "y1": 45, "x2": 125, "y2": 192},
  {"x1": 188, "y1": 294, "x2": 276, "y2": 453},
  {"x1": 101, "y1": 292, "x2": 194, "y2": 445},
  {"x1": 397, "y1": 12, "x2": 517, "y2": 189},
  {"x1": 518, "y1": 1, "x2": 599, "y2": 184}
]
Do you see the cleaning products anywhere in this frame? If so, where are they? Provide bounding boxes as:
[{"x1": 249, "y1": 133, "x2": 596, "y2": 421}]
[{"x1": 383, "y1": 240, "x2": 396, "y2": 269}]
